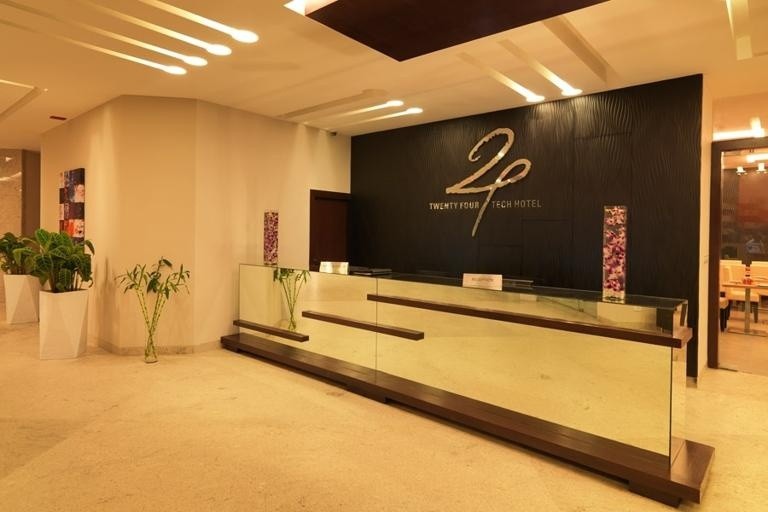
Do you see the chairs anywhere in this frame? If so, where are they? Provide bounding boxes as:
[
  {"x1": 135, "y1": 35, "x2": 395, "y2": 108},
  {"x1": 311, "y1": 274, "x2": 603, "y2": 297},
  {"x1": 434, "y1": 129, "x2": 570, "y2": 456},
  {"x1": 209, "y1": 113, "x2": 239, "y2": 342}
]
[{"x1": 719, "y1": 257, "x2": 768, "y2": 333}]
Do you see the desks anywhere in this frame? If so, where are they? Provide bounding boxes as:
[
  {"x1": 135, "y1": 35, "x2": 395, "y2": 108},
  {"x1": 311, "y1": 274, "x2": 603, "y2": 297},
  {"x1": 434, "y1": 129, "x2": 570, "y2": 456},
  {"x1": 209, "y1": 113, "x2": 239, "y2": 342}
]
[{"x1": 721, "y1": 283, "x2": 768, "y2": 338}]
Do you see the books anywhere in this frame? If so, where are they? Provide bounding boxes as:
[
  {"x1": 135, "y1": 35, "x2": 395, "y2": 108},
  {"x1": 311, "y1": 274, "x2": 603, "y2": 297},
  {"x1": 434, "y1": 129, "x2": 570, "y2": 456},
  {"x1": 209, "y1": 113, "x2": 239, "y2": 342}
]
[{"x1": 350, "y1": 268, "x2": 392, "y2": 276}]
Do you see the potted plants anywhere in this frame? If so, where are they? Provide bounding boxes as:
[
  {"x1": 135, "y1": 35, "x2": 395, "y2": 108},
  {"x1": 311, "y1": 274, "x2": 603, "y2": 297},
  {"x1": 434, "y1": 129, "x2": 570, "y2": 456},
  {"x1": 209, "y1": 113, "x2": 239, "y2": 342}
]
[
  {"x1": 273, "y1": 267, "x2": 311, "y2": 332},
  {"x1": 0, "y1": 229, "x2": 95, "y2": 359},
  {"x1": 114, "y1": 256, "x2": 189, "y2": 364}
]
